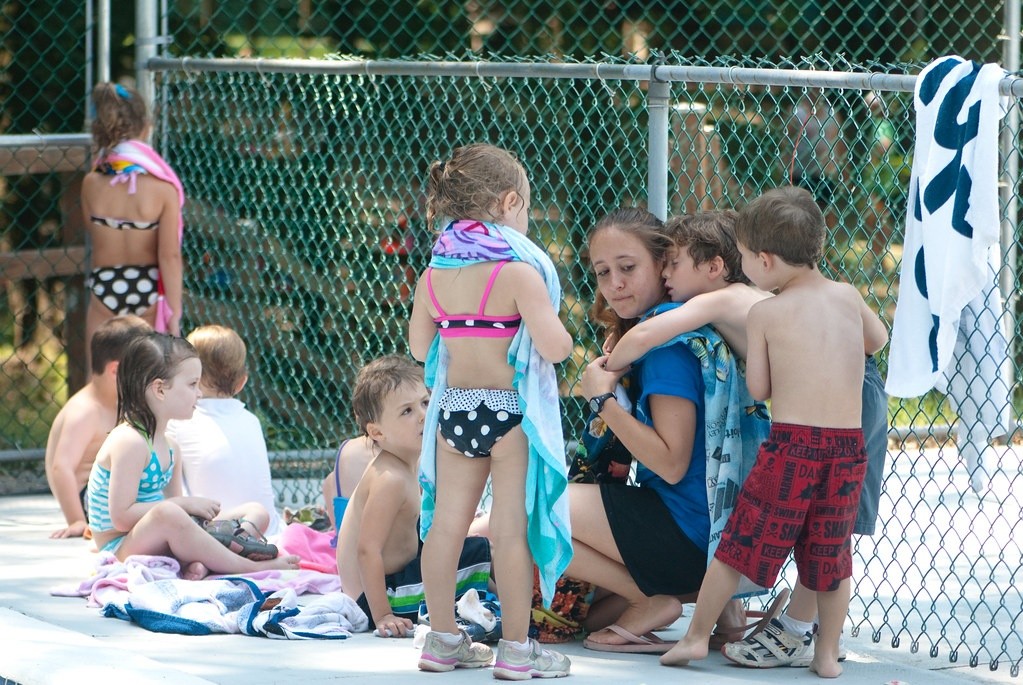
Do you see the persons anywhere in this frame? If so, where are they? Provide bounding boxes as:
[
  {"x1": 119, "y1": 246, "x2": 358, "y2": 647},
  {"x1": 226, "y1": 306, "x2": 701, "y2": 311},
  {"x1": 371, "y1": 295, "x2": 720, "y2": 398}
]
[
  {"x1": 781, "y1": 89, "x2": 843, "y2": 252},
  {"x1": 42, "y1": 316, "x2": 298, "y2": 580},
  {"x1": 858, "y1": 93, "x2": 896, "y2": 274},
  {"x1": 323, "y1": 144, "x2": 890, "y2": 679},
  {"x1": 82, "y1": 81, "x2": 182, "y2": 384}
]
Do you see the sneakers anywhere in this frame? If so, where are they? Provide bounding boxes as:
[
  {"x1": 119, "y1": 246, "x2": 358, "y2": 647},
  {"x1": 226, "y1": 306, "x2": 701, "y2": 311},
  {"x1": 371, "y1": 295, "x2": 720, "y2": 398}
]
[
  {"x1": 814, "y1": 623, "x2": 847, "y2": 661},
  {"x1": 720, "y1": 617, "x2": 816, "y2": 667},
  {"x1": 190, "y1": 514, "x2": 207, "y2": 528},
  {"x1": 203, "y1": 518, "x2": 278, "y2": 561},
  {"x1": 418, "y1": 629, "x2": 493, "y2": 672},
  {"x1": 492, "y1": 635, "x2": 570, "y2": 680}
]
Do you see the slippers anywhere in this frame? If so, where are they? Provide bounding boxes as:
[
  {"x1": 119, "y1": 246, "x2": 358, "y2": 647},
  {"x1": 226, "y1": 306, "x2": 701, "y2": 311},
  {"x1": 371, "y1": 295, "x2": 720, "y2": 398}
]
[
  {"x1": 709, "y1": 588, "x2": 790, "y2": 649},
  {"x1": 583, "y1": 624, "x2": 680, "y2": 653}
]
